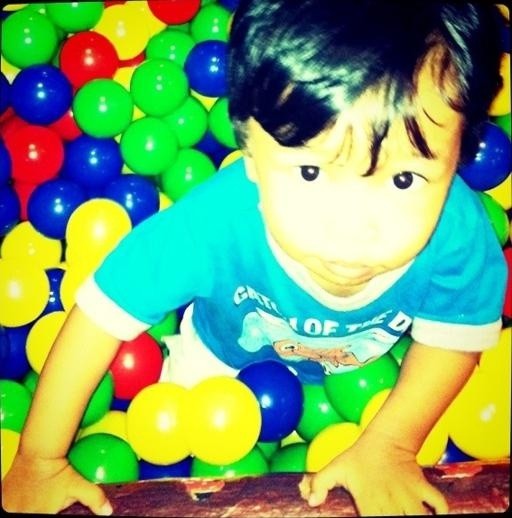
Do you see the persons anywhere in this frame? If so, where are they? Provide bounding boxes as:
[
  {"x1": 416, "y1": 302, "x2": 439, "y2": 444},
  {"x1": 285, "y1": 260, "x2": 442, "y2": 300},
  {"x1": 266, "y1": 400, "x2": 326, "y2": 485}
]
[{"x1": 1, "y1": 2, "x2": 509, "y2": 518}]
